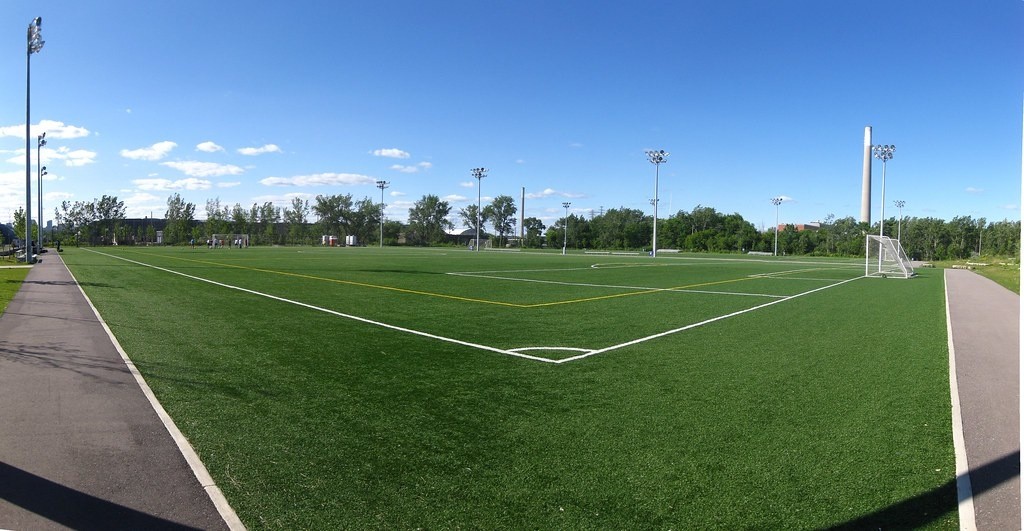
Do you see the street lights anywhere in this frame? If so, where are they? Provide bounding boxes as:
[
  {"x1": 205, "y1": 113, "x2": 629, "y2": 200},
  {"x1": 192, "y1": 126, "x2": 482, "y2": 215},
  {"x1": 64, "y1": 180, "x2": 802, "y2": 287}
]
[
  {"x1": 645, "y1": 150, "x2": 670, "y2": 257},
  {"x1": 376, "y1": 180, "x2": 391, "y2": 248},
  {"x1": 893, "y1": 200, "x2": 905, "y2": 261},
  {"x1": 562, "y1": 202, "x2": 572, "y2": 250},
  {"x1": 770, "y1": 198, "x2": 782, "y2": 257},
  {"x1": 470, "y1": 168, "x2": 489, "y2": 251},
  {"x1": 25, "y1": 16, "x2": 46, "y2": 263},
  {"x1": 871, "y1": 143, "x2": 895, "y2": 275},
  {"x1": 37, "y1": 133, "x2": 47, "y2": 255}
]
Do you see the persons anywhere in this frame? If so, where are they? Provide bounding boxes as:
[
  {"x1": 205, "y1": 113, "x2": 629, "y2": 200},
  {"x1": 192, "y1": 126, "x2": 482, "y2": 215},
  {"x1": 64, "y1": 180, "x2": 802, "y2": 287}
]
[
  {"x1": 190, "y1": 237, "x2": 195, "y2": 249},
  {"x1": 207, "y1": 238, "x2": 247, "y2": 249},
  {"x1": 55, "y1": 239, "x2": 61, "y2": 252}
]
